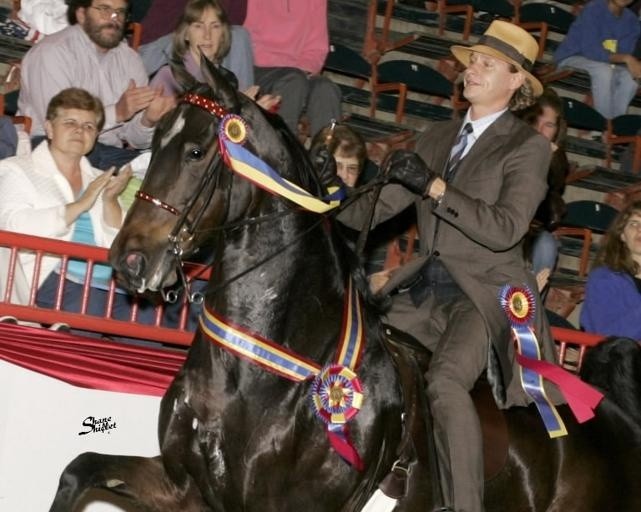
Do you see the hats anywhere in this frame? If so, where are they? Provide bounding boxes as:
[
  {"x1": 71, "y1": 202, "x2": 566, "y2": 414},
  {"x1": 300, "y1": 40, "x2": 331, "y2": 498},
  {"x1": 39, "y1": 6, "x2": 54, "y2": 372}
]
[{"x1": 449, "y1": 20, "x2": 543, "y2": 98}]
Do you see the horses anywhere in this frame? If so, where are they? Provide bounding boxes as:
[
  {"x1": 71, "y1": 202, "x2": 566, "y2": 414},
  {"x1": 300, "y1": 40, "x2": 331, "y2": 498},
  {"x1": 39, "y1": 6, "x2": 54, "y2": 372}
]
[{"x1": 49, "y1": 44, "x2": 641, "y2": 512}]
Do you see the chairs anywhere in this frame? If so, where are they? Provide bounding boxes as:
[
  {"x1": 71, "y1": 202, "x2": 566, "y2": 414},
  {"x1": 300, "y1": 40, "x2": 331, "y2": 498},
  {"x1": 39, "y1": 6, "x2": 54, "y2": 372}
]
[{"x1": 0, "y1": 1, "x2": 641, "y2": 369}]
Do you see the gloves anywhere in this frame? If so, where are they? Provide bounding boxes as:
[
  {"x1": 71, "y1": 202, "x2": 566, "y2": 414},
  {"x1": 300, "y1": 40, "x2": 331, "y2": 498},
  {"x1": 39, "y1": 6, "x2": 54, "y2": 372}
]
[{"x1": 384, "y1": 147, "x2": 436, "y2": 200}]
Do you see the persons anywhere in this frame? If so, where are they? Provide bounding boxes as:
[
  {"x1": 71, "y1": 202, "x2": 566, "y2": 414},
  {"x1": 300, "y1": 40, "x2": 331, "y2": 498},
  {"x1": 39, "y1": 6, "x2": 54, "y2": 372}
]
[
  {"x1": 553, "y1": 0, "x2": 641, "y2": 139},
  {"x1": 580, "y1": 202, "x2": 641, "y2": 342},
  {"x1": 16, "y1": 1, "x2": 179, "y2": 174},
  {"x1": 312, "y1": 124, "x2": 369, "y2": 187},
  {"x1": 310, "y1": 19, "x2": 555, "y2": 511},
  {"x1": 241, "y1": 2, "x2": 344, "y2": 152},
  {"x1": 1, "y1": 88, "x2": 170, "y2": 355},
  {"x1": 517, "y1": 87, "x2": 573, "y2": 299},
  {"x1": 151, "y1": 1, "x2": 285, "y2": 114}
]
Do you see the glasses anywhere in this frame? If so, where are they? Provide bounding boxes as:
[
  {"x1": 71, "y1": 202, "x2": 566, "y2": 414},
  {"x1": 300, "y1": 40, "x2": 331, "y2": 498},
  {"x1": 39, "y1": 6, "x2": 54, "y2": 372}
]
[
  {"x1": 335, "y1": 161, "x2": 365, "y2": 176},
  {"x1": 54, "y1": 117, "x2": 101, "y2": 135},
  {"x1": 87, "y1": 5, "x2": 131, "y2": 26}
]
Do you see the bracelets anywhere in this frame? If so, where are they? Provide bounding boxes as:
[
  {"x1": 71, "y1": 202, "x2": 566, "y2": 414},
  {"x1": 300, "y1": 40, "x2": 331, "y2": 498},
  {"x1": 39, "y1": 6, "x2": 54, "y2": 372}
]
[{"x1": 436, "y1": 191, "x2": 446, "y2": 201}]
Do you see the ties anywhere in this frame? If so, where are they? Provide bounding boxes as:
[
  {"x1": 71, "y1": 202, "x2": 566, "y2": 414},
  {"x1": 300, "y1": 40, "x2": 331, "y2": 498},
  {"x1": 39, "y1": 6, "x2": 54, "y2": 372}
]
[{"x1": 441, "y1": 123, "x2": 473, "y2": 183}]
